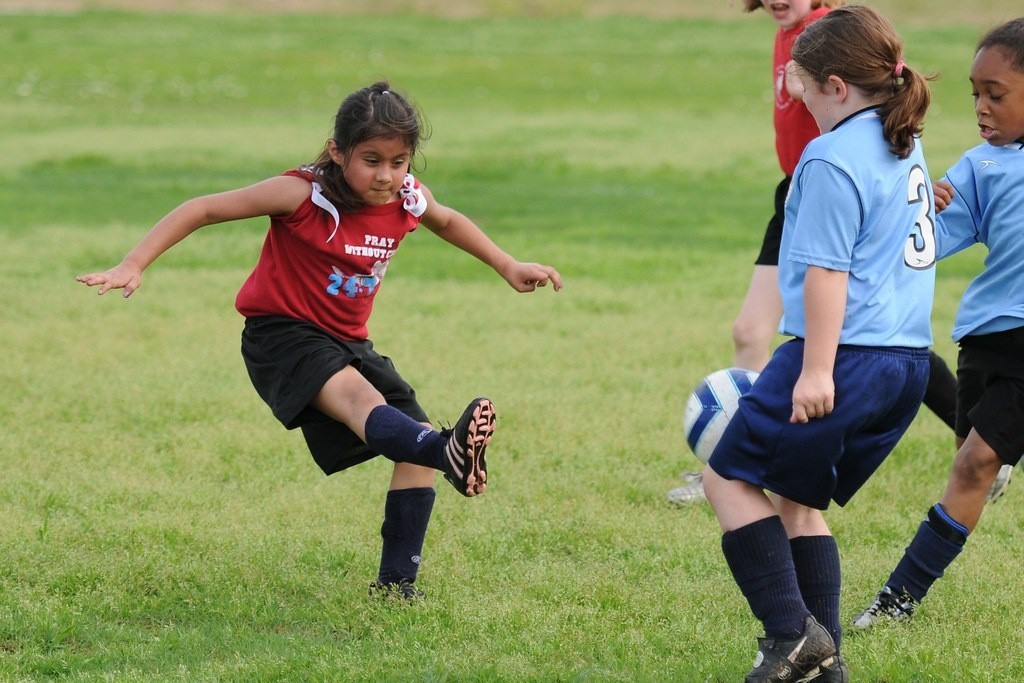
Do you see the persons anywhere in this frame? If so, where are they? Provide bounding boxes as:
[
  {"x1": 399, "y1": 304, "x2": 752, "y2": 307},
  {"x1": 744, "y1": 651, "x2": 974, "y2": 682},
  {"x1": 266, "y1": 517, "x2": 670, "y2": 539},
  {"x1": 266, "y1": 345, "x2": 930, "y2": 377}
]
[
  {"x1": 76, "y1": 83, "x2": 564, "y2": 598},
  {"x1": 664, "y1": 0, "x2": 1024, "y2": 683}
]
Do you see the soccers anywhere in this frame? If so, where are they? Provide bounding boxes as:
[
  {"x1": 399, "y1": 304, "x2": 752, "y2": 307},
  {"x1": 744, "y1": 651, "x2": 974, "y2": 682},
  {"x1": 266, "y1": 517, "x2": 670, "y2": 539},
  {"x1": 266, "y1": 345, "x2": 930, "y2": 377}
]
[{"x1": 682, "y1": 366, "x2": 761, "y2": 465}]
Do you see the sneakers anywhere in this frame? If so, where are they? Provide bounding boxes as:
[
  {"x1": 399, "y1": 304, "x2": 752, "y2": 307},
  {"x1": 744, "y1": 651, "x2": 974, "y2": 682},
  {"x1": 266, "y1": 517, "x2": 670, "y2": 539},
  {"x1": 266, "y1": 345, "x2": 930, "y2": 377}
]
[
  {"x1": 369, "y1": 580, "x2": 427, "y2": 607},
  {"x1": 850, "y1": 583, "x2": 916, "y2": 629},
  {"x1": 668, "y1": 473, "x2": 708, "y2": 505},
  {"x1": 987, "y1": 463, "x2": 1014, "y2": 504},
  {"x1": 437, "y1": 397, "x2": 497, "y2": 497},
  {"x1": 744, "y1": 614, "x2": 848, "y2": 682}
]
